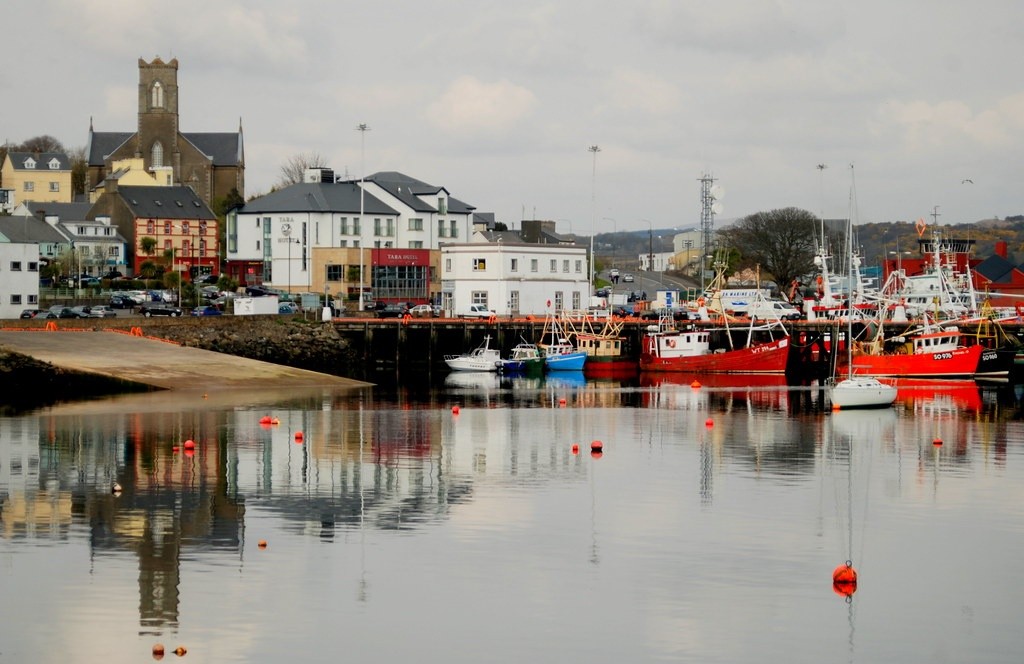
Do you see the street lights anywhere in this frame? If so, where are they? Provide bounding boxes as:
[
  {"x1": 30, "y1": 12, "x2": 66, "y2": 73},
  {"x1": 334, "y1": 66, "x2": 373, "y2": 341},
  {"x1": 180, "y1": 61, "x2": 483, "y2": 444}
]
[
  {"x1": 587, "y1": 144, "x2": 601, "y2": 301},
  {"x1": 355, "y1": 122, "x2": 371, "y2": 311}
]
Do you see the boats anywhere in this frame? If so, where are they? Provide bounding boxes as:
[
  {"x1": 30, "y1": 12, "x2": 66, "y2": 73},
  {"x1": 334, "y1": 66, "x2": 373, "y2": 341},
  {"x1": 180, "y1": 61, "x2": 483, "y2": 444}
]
[
  {"x1": 640, "y1": 234, "x2": 790, "y2": 373},
  {"x1": 502, "y1": 366, "x2": 588, "y2": 389},
  {"x1": 574, "y1": 309, "x2": 636, "y2": 370},
  {"x1": 501, "y1": 340, "x2": 544, "y2": 369},
  {"x1": 443, "y1": 367, "x2": 499, "y2": 391},
  {"x1": 537, "y1": 305, "x2": 587, "y2": 370},
  {"x1": 444, "y1": 334, "x2": 501, "y2": 371},
  {"x1": 809, "y1": 185, "x2": 1024, "y2": 378}
]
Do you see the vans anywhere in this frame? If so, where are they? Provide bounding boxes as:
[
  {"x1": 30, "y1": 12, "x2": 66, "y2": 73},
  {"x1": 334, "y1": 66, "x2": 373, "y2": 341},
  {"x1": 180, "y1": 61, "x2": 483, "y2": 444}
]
[{"x1": 748, "y1": 301, "x2": 800, "y2": 321}]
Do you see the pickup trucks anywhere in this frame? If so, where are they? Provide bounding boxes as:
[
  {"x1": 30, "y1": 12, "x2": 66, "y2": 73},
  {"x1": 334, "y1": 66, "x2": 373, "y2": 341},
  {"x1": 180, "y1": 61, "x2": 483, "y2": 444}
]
[{"x1": 456, "y1": 304, "x2": 495, "y2": 318}]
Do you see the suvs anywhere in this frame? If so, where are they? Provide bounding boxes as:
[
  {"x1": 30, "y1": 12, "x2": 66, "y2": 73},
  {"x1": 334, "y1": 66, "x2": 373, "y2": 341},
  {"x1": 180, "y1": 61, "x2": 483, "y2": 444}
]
[{"x1": 140, "y1": 300, "x2": 182, "y2": 318}]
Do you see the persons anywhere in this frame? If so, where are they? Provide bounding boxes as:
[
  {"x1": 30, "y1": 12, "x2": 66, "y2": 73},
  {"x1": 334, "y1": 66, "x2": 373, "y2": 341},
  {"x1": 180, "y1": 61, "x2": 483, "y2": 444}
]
[
  {"x1": 403, "y1": 309, "x2": 412, "y2": 316},
  {"x1": 610, "y1": 275, "x2": 620, "y2": 284},
  {"x1": 631, "y1": 291, "x2": 647, "y2": 301}
]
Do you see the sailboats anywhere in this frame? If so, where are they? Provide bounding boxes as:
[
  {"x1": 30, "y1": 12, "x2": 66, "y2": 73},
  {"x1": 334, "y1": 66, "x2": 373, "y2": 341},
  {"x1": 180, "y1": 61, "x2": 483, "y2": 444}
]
[{"x1": 821, "y1": 171, "x2": 899, "y2": 409}]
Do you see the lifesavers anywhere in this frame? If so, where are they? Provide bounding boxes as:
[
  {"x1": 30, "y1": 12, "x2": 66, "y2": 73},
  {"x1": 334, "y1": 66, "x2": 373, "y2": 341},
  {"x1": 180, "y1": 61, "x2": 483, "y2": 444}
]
[
  {"x1": 916, "y1": 347, "x2": 924, "y2": 355},
  {"x1": 670, "y1": 340, "x2": 676, "y2": 349}
]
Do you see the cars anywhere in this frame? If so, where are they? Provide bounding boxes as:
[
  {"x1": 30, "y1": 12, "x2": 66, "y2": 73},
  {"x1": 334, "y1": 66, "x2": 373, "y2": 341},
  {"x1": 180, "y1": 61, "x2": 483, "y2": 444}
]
[
  {"x1": 365, "y1": 299, "x2": 443, "y2": 319},
  {"x1": 642, "y1": 306, "x2": 701, "y2": 321},
  {"x1": 40, "y1": 270, "x2": 176, "y2": 308},
  {"x1": 21, "y1": 305, "x2": 117, "y2": 319},
  {"x1": 595, "y1": 268, "x2": 647, "y2": 301},
  {"x1": 189, "y1": 272, "x2": 299, "y2": 316}
]
